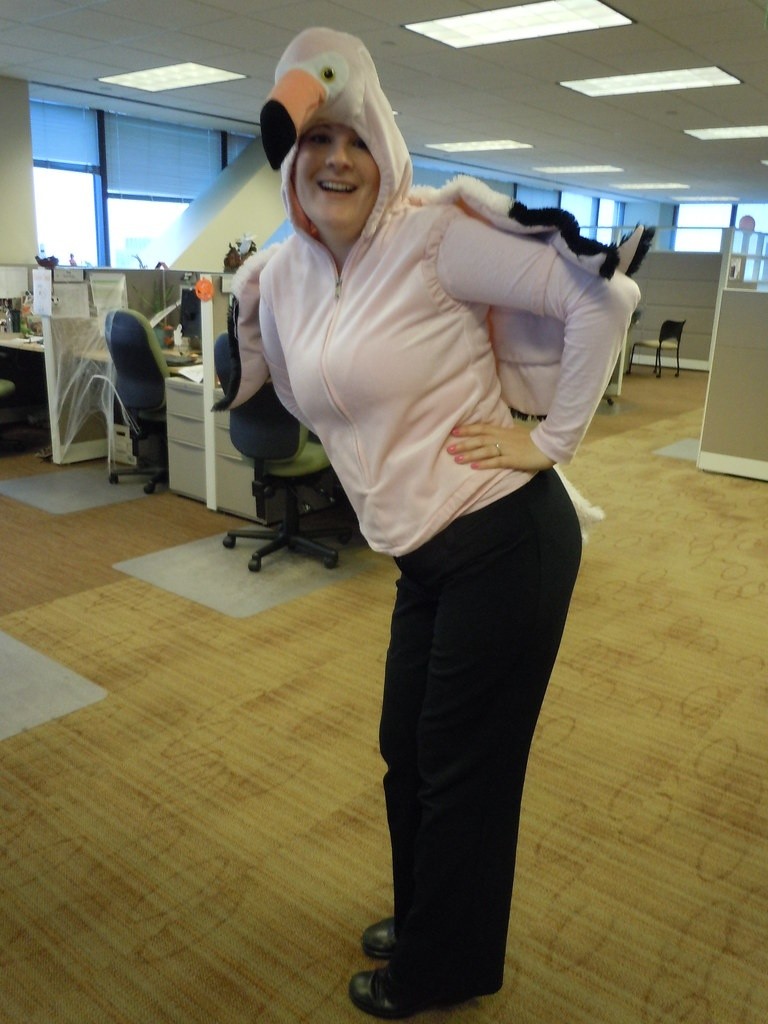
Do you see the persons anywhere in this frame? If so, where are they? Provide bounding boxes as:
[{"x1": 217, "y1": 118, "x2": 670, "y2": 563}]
[{"x1": 212, "y1": 90, "x2": 643, "y2": 1023}]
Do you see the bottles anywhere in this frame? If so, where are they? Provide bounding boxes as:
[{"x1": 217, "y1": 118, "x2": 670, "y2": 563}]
[
  {"x1": 11, "y1": 308, "x2": 20, "y2": 333},
  {"x1": 20, "y1": 308, "x2": 32, "y2": 338},
  {"x1": 39, "y1": 244, "x2": 45, "y2": 259}
]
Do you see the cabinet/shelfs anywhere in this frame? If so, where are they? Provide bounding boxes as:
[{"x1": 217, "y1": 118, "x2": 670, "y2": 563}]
[
  {"x1": 212, "y1": 387, "x2": 336, "y2": 526},
  {"x1": 163, "y1": 377, "x2": 207, "y2": 505}
]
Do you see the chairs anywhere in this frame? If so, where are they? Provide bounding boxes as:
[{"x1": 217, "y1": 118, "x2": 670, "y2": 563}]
[
  {"x1": 214, "y1": 331, "x2": 353, "y2": 573},
  {"x1": 627, "y1": 319, "x2": 685, "y2": 378},
  {"x1": 104, "y1": 307, "x2": 171, "y2": 495},
  {"x1": 0, "y1": 379, "x2": 26, "y2": 452}
]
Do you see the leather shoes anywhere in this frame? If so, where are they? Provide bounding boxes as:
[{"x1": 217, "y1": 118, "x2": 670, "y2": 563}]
[
  {"x1": 349, "y1": 964, "x2": 476, "y2": 1018},
  {"x1": 361, "y1": 917, "x2": 397, "y2": 959}
]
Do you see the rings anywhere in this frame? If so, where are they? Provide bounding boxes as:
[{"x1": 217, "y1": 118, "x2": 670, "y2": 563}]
[{"x1": 494, "y1": 443, "x2": 502, "y2": 456}]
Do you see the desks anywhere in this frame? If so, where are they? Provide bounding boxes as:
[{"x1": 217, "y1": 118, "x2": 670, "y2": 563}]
[
  {"x1": 74, "y1": 347, "x2": 203, "y2": 376},
  {"x1": 0, "y1": 330, "x2": 45, "y2": 354}
]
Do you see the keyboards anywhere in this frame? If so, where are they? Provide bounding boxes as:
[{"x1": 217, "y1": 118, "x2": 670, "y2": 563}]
[{"x1": 163, "y1": 354, "x2": 197, "y2": 367}]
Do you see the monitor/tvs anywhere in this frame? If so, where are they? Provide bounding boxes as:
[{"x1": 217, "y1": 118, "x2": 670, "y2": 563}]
[{"x1": 178, "y1": 286, "x2": 202, "y2": 357}]
